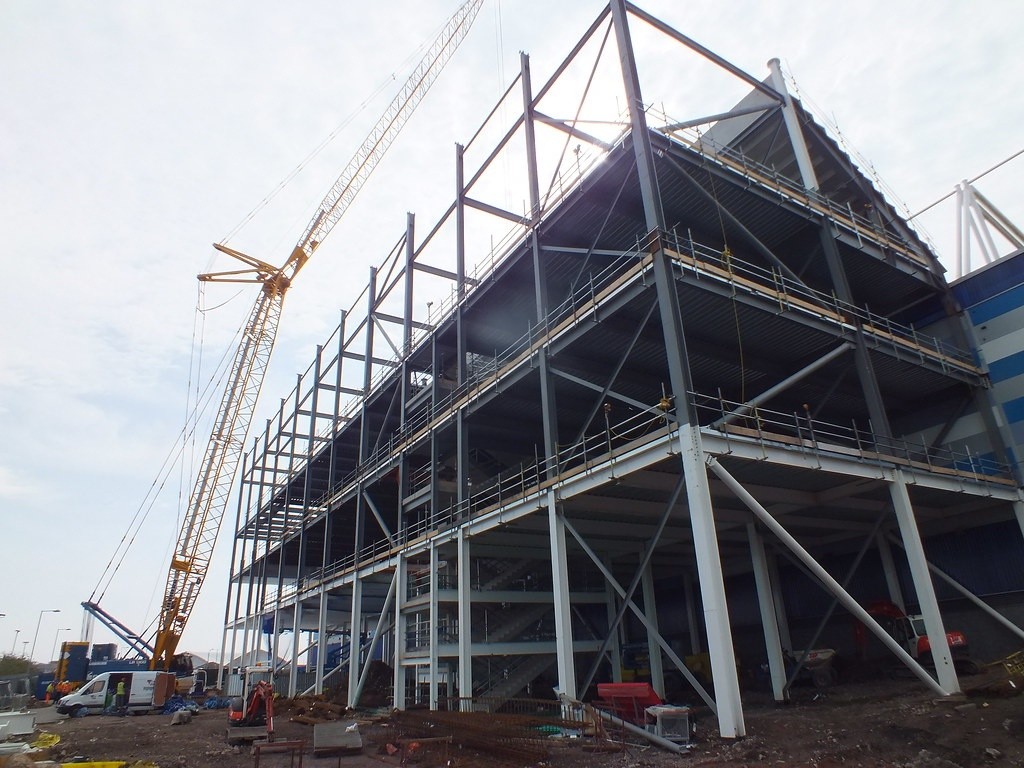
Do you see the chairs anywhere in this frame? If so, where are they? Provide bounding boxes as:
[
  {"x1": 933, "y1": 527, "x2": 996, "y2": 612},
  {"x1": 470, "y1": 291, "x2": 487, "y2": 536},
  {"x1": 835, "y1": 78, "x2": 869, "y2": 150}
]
[{"x1": 983, "y1": 651, "x2": 1024, "y2": 690}]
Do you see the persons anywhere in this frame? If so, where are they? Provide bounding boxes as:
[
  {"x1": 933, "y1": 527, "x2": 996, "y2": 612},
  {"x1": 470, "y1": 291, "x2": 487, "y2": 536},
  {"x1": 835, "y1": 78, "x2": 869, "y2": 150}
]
[
  {"x1": 115, "y1": 677, "x2": 128, "y2": 708},
  {"x1": 45, "y1": 679, "x2": 71, "y2": 704}
]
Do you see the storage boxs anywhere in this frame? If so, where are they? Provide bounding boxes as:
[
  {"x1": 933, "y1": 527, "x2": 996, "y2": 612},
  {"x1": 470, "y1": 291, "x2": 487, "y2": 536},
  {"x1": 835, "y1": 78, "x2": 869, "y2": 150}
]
[{"x1": 644, "y1": 709, "x2": 689, "y2": 741}]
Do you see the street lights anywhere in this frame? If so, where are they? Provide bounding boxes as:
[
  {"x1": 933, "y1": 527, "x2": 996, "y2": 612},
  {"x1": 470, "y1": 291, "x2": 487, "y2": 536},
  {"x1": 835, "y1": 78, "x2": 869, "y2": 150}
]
[
  {"x1": 52, "y1": 628, "x2": 72, "y2": 661},
  {"x1": 10, "y1": 629, "x2": 21, "y2": 655},
  {"x1": 27, "y1": 609, "x2": 62, "y2": 674},
  {"x1": 23, "y1": 641, "x2": 30, "y2": 658}
]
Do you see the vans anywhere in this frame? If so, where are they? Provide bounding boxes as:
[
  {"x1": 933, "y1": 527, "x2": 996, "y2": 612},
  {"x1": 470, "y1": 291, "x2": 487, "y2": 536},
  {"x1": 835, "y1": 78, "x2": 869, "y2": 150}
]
[{"x1": 55, "y1": 669, "x2": 164, "y2": 718}]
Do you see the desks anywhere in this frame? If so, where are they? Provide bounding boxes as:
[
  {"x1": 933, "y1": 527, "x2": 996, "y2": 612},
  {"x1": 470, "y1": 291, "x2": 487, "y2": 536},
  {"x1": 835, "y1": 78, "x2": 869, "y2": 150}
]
[{"x1": 254, "y1": 740, "x2": 305, "y2": 768}]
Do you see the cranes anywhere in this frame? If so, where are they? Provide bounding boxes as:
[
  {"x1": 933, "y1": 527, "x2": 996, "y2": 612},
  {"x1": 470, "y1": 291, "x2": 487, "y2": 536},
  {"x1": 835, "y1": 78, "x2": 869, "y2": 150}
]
[{"x1": 149, "y1": 0, "x2": 501, "y2": 671}]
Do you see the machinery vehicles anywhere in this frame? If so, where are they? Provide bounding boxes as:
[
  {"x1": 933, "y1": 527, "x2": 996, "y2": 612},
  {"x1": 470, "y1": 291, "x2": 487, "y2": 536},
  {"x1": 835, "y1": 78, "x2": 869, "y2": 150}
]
[
  {"x1": 853, "y1": 604, "x2": 989, "y2": 682},
  {"x1": 225, "y1": 662, "x2": 275, "y2": 746}
]
